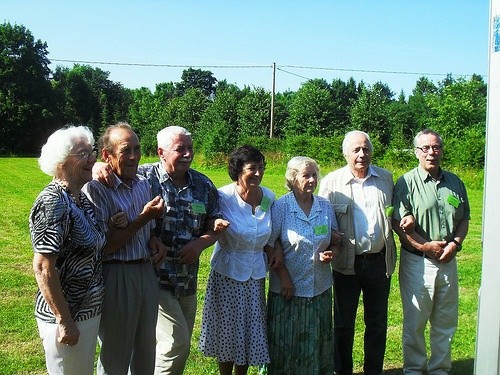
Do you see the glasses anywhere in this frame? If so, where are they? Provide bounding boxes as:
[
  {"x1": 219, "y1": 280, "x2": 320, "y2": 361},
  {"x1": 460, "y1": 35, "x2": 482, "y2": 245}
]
[
  {"x1": 415, "y1": 145, "x2": 443, "y2": 153},
  {"x1": 68, "y1": 150, "x2": 98, "y2": 159}
]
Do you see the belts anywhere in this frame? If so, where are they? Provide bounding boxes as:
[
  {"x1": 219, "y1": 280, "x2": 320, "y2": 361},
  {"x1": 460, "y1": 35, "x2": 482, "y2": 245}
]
[
  {"x1": 106, "y1": 258, "x2": 145, "y2": 264},
  {"x1": 401, "y1": 245, "x2": 430, "y2": 258}
]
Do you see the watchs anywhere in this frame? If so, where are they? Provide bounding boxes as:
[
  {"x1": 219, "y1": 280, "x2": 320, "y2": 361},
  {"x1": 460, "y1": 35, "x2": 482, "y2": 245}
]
[{"x1": 450, "y1": 239, "x2": 462, "y2": 252}]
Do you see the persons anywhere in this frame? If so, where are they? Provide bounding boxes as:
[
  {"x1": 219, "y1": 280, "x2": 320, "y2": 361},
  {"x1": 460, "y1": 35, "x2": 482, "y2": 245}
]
[
  {"x1": 263, "y1": 155, "x2": 339, "y2": 375},
  {"x1": 82, "y1": 122, "x2": 167, "y2": 375},
  {"x1": 317, "y1": 130, "x2": 416, "y2": 375},
  {"x1": 392, "y1": 130, "x2": 471, "y2": 375},
  {"x1": 29, "y1": 125, "x2": 129, "y2": 375},
  {"x1": 203, "y1": 144, "x2": 285, "y2": 375},
  {"x1": 92, "y1": 126, "x2": 221, "y2": 375}
]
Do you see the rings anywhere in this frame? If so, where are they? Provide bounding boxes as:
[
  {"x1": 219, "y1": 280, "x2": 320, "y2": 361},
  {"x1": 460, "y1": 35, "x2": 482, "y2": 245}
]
[{"x1": 111, "y1": 217, "x2": 114, "y2": 221}]
